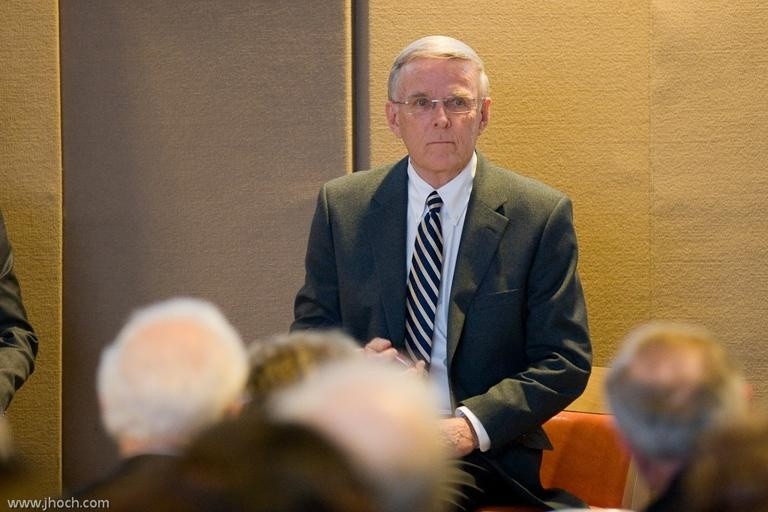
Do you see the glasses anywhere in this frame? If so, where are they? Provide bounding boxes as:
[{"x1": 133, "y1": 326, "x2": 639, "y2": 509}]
[{"x1": 390, "y1": 95, "x2": 480, "y2": 114}]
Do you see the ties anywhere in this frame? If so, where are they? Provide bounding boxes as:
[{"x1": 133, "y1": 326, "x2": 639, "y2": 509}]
[{"x1": 399, "y1": 192, "x2": 443, "y2": 373}]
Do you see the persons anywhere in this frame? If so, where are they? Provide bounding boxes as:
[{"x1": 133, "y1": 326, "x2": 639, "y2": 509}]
[
  {"x1": 287, "y1": 35, "x2": 593, "y2": 511},
  {"x1": 44, "y1": 298, "x2": 475, "y2": 511},
  {"x1": 600, "y1": 320, "x2": 767, "y2": 511},
  {"x1": 0, "y1": 213, "x2": 39, "y2": 416}
]
